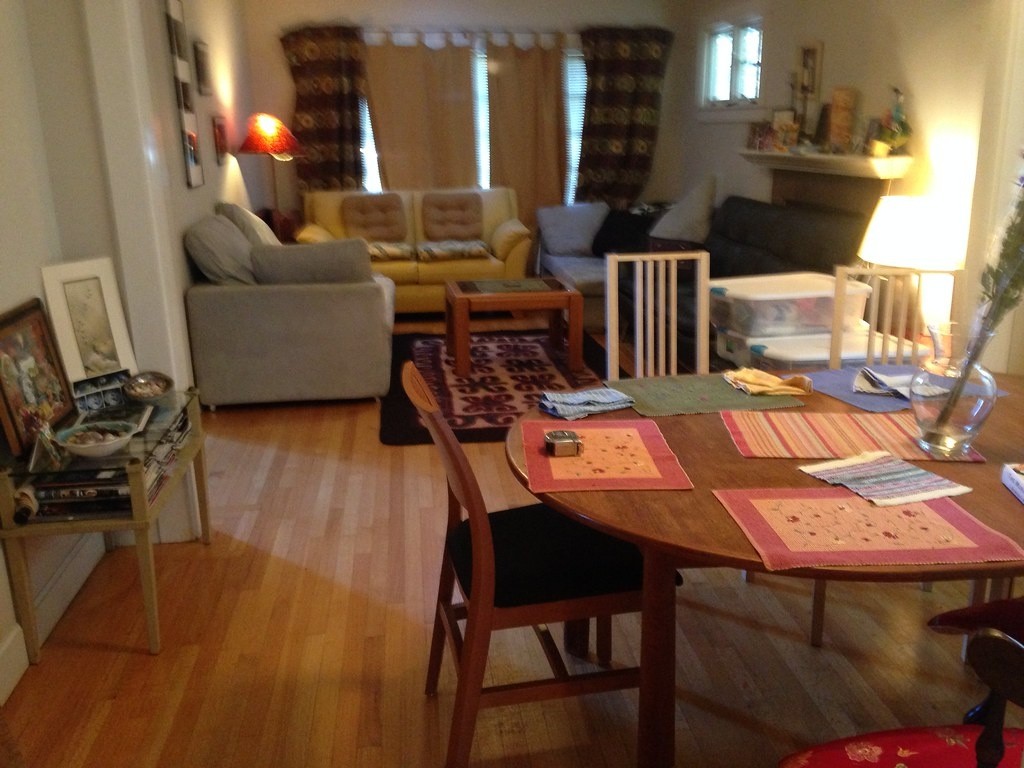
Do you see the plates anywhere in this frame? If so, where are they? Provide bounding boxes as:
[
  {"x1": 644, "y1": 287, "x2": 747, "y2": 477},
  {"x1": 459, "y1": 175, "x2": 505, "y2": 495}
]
[{"x1": 120, "y1": 370, "x2": 175, "y2": 401}]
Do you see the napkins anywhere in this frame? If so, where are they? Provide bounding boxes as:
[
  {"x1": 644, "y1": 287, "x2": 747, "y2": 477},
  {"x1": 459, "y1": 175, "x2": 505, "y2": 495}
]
[
  {"x1": 853, "y1": 365, "x2": 949, "y2": 398},
  {"x1": 539, "y1": 387, "x2": 636, "y2": 421},
  {"x1": 722, "y1": 366, "x2": 815, "y2": 396}
]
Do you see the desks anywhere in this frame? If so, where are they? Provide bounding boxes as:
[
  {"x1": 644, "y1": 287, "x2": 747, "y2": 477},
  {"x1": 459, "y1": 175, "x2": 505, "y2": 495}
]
[
  {"x1": 505, "y1": 371, "x2": 1024, "y2": 768},
  {"x1": 1, "y1": 387, "x2": 216, "y2": 664}
]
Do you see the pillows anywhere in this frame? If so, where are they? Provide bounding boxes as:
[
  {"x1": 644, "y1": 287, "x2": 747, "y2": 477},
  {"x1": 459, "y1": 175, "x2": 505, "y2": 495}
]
[{"x1": 649, "y1": 179, "x2": 717, "y2": 242}]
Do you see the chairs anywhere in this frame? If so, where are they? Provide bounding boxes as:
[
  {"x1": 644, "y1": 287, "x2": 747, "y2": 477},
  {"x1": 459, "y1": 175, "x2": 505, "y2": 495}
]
[
  {"x1": 398, "y1": 361, "x2": 1024, "y2": 768},
  {"x1": 806, "y1": 268, "x2": 988, "y2": 667},
  {"x1": 606, "y1": 253, "x2": 762, "y2": 585}
]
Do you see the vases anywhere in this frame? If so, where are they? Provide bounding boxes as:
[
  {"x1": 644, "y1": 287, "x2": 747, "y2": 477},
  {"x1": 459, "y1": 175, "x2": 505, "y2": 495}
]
[{"x1": 910, "y1": 323, "x2": 999, "y2": 457}]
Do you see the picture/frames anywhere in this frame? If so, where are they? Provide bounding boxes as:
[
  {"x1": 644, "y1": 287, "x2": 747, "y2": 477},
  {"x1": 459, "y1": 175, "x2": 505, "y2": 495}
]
[
  {"x1": 0, "y1": 258, "x2": 143, "y2": 459},
  {"x1": 747, "y1": 40, "x2": 823, "y2": 151},
  {"x1": 166, "y1": 0, "x2": 229, "y2": 190}
]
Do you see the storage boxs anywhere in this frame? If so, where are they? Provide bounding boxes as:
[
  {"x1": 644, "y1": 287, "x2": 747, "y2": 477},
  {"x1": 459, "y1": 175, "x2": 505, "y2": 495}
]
[
  {"x1": 706, "y1": 271, "x2": 916, "y2": 362},
  {"x1": 1002, "y1": 461, "x2": 1024, "y2": 504}
]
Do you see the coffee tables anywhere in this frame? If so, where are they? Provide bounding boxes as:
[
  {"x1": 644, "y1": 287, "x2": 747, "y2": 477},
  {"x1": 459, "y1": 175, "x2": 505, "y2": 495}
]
[{"x1": 443, "y1": 276, "x2": 586, "y2": 375}]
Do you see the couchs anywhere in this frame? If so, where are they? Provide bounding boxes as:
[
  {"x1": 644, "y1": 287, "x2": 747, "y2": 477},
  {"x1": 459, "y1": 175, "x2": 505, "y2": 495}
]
[
  {"x1": 295, "y1": 186, "x2": 531, "y2": 316},
  {"x1": 534, "y1": 201, "x2": 872, "y2": 368},
  {"x1": 185, "y1": 202, "x2": 395, "y2": 413}
]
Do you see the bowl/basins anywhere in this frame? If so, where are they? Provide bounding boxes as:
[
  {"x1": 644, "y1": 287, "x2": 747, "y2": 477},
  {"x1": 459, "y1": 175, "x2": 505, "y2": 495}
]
[{"x1": 52, "y1": 421, "x2": 139, "y2": 457}]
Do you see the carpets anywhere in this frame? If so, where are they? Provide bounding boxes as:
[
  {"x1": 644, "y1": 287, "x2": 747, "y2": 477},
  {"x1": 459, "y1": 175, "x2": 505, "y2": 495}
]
[{"x1": 381, "y1": 330, "x2": 631, "y2": 446}]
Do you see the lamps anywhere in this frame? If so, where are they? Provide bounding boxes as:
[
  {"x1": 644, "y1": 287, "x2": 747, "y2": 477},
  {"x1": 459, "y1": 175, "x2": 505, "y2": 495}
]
[
  {"x1": 236, "y1": 111, "x2": 307, "y2": 240},
  {"x1": 858, "y1": 193, "x2": 967, "y2": 351}
]
